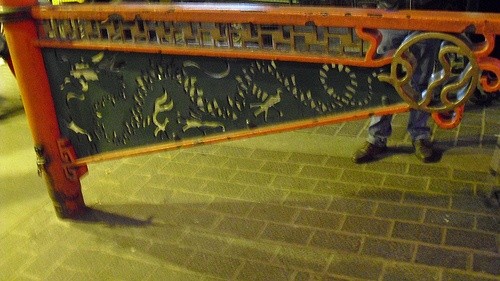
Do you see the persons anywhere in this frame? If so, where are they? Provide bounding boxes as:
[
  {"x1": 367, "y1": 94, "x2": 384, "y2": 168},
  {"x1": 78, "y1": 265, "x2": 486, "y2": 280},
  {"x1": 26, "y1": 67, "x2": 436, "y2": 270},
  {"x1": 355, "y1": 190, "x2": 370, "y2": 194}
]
[{"x1": 351, "y1": 0, "x2": 446, "y2": 163}]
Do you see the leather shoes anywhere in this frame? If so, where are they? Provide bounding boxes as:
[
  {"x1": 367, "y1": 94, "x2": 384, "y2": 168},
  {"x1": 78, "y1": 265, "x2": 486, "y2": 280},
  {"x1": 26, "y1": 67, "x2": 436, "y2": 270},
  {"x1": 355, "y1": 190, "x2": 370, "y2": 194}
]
[
  {"x1": 412, "y1": 136, "x2": 435, "y2": 162},
  {"x1": 351, "y1": 140, "x2": 386, "y2": 163}
]
